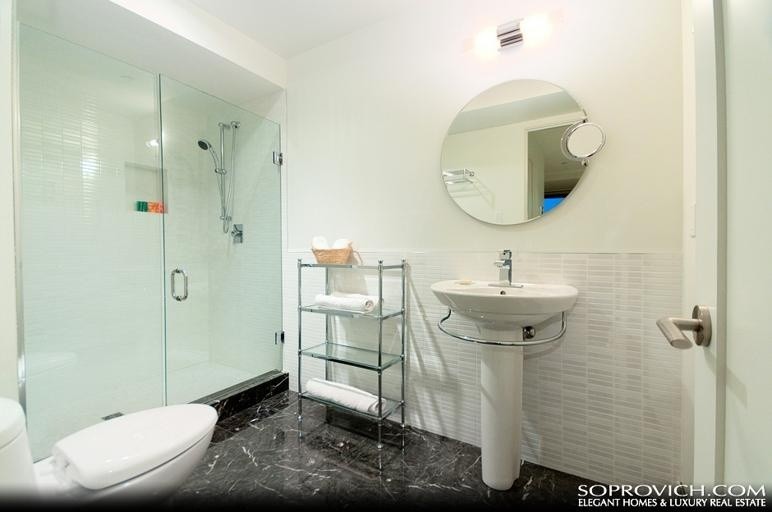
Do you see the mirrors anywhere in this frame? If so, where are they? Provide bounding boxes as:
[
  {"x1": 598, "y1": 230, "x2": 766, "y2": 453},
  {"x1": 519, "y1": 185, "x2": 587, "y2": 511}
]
[
  {"x1": 558, "y1": 117, "x2": 605, "y2": 163},
  {"x1": 440, "y1": 77, "x2": 593, "y2": 229}
]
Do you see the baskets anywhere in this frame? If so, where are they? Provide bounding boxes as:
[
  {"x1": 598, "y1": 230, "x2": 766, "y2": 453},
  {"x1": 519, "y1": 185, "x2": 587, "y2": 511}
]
[{"x1": 313, "y1": 249, "x2": 352, "y2": 264}]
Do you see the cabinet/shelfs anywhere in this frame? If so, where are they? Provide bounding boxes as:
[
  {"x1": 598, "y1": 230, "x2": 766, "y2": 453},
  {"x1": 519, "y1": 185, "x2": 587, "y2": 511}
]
[{"x1": 292, "y1": 259, "x2": 409, "y2": 449}]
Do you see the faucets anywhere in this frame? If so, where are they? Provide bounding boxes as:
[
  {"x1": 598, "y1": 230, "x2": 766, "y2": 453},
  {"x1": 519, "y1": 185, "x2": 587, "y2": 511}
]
[{"x1": 493, "y1": 248, "x2": 512, "y2": 284}]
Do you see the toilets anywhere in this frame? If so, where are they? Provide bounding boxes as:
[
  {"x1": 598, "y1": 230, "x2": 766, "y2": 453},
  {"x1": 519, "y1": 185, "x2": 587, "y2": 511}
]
[{"x1": 0, "y1": 398, "x2": 218, "y2": 512}]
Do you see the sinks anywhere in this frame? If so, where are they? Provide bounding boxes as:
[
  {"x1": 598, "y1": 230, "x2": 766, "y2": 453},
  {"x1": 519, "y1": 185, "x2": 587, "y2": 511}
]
[{"x1": 430, "y1": 277, "x2": 578, "y2": 328}]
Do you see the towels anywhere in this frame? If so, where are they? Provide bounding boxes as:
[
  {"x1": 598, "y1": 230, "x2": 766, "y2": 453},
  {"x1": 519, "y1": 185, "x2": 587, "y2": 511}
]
[
  {"x1": 313, "y1": 290, "x2": 381, "y2": 314},
  {"x1": 311, "y1": 236, "x2": 351, "y2": 248},
  {"x1": 304, "y1": 376, "x2": 388, "y2": 417}
]
